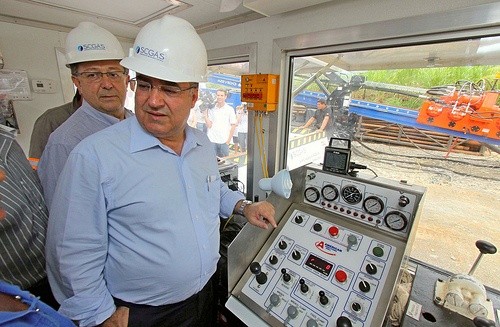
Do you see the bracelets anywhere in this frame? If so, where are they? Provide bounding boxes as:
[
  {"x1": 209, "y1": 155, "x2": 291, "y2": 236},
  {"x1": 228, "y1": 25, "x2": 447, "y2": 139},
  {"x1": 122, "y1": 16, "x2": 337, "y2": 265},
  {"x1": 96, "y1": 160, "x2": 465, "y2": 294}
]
[{"x1": 238, "y1": 200, "x2": 252, "y2": 216}]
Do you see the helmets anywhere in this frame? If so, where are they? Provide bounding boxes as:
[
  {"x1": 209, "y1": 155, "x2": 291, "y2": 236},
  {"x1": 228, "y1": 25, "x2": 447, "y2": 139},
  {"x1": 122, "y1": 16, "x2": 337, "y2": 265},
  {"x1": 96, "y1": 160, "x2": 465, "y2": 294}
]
[
  {"x1": 117, "y1": 14, "x2": 208, "y2": 83},
  {"x1": 65, "y1": 21, "x2": 124, "y2": 65}
]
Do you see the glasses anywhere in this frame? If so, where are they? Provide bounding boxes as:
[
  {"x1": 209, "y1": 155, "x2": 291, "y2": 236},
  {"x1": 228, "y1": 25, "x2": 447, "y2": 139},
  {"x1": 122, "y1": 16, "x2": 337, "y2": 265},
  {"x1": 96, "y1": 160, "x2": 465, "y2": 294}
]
[
  {"x1": 128, "y1": 76, "x2": 196, "y2": 98},
  {"x1": 73, "y1": 71, "x2": 125, "y2": 81}
]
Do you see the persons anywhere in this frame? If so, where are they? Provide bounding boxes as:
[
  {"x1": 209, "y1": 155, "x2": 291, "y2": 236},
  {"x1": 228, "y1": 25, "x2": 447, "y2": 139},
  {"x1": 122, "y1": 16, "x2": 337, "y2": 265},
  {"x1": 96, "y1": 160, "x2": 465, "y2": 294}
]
[
  {"x1": 28, "y1": 87, "x2": 84, "y2": 159},
  {"x1": 44, "y1": 14, "x2": 277, "y2": 327},
  {"x1": 36, "y1": 19, "x2": 136, "y2": 214},
  {"x1": 0, "y1": 279, "x2": 78, "y2": 327},
  {"x1": 186, "y1": 89, "x2": 248, "y2": 159},
  {"x1": 298, "y1": 100, "x2": 333, "y2": 134},
  {"x1": 0, "y1": 125, "x2": 61, "y2": 311}
]
